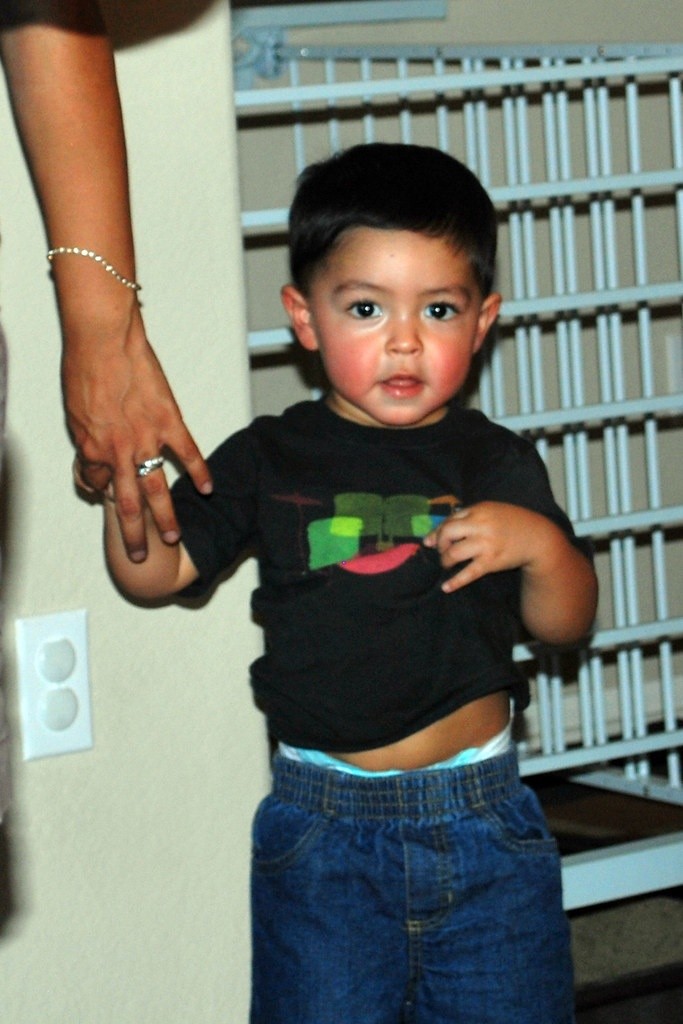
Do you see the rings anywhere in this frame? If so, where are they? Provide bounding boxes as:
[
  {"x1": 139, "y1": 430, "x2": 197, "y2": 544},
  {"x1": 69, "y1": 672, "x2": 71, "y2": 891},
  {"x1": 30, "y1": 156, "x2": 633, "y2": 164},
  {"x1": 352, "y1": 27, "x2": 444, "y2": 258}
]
[{"x1": 132, "y1": 452, "x2": 166, "y2": 478}]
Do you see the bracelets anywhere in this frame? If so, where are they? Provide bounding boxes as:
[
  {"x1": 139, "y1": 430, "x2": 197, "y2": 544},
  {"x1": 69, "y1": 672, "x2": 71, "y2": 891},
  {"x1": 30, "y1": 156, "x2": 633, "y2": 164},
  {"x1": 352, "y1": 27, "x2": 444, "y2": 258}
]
[{"x1": 46, "y1": 247, "x2": 144, "y2": 292}]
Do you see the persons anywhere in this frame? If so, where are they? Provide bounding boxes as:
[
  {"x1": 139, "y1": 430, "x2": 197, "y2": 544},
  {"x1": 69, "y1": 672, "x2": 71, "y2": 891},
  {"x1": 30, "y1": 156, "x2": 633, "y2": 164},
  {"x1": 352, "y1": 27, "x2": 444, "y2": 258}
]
[
  {"x1": 93, "y1": 140, "x2": 601, "y2": 1024},
  {"x1": 0, "y1": 0, "x2": 220, "y2": 565}
]
